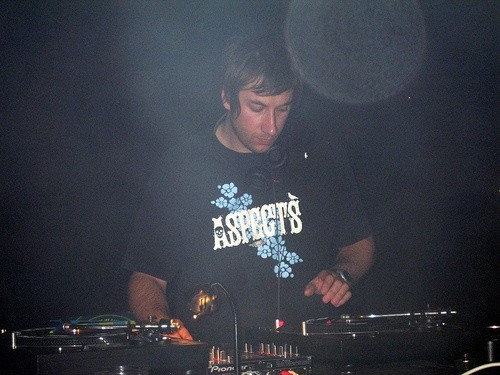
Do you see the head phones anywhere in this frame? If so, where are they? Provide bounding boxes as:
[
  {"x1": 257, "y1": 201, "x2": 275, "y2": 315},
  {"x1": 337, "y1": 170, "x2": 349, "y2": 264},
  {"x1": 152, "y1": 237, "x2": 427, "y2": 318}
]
[{"x1": 263, "y1": 144, "x2": 288, "y2": 170}]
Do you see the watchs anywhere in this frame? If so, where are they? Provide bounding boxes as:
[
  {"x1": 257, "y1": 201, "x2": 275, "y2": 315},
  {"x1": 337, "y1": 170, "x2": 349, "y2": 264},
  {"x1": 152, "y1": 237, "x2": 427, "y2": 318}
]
[{"x1": 329, "y1": 264, "x2": 355, "y2": 292}]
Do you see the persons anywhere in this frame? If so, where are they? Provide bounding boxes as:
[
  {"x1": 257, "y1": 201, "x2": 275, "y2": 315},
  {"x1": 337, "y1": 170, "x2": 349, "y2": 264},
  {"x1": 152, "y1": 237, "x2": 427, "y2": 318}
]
[{"x1": 121, "y1": 35, "x2": 377, "y2": 344}]
